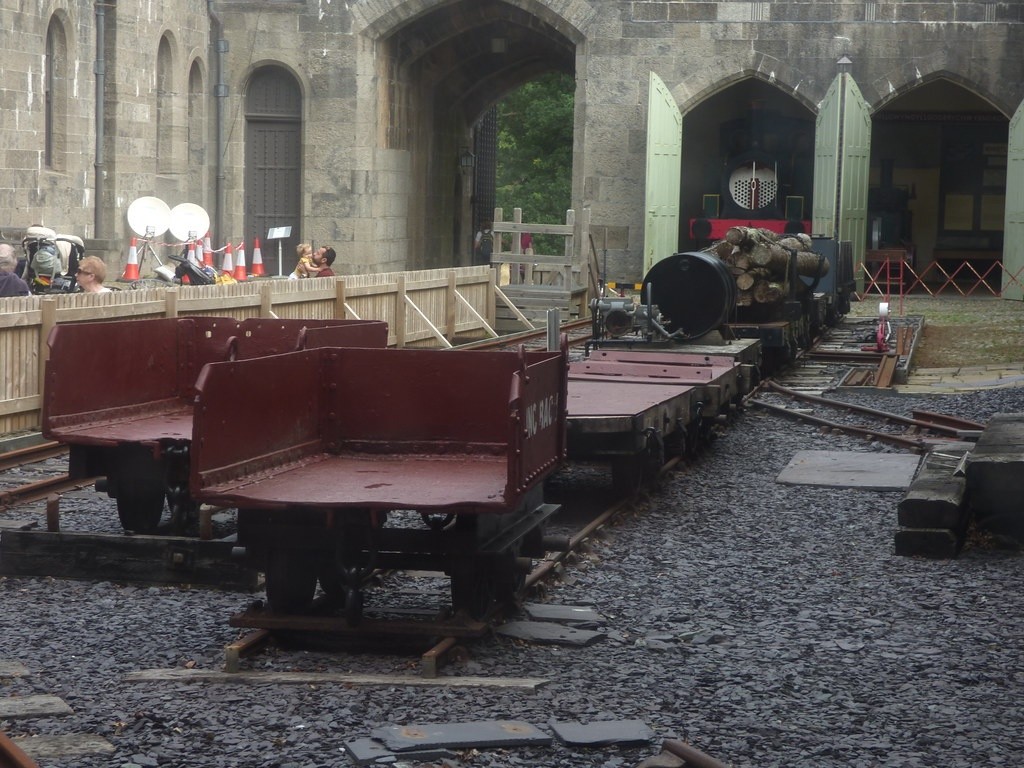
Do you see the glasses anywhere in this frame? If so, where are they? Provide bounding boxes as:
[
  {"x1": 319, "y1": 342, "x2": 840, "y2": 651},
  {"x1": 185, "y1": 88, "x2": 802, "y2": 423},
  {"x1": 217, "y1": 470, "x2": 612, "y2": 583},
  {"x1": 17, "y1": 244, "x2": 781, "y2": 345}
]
[{"x1": 76, "y1": 267, "x2": 93, "y2": 275}]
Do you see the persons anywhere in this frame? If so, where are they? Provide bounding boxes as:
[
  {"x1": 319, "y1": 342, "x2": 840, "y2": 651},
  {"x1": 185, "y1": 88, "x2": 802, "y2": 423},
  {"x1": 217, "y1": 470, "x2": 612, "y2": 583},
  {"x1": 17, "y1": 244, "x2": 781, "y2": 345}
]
[
  {"x1": 475, "y1": 220, "x2": 506, "y2": 264},
  {"x1": 0, "y1": 243, "x2": 30, "y2": 296},
  {"x1": 73, "y1": 253, "x2": 109, "y2": 292},
  {"x1": 514, "y1": 221, "x2": 533, "y2": 255},
  {"x1": 288, "y1": 242, "x2": 334, "y2": 279}
]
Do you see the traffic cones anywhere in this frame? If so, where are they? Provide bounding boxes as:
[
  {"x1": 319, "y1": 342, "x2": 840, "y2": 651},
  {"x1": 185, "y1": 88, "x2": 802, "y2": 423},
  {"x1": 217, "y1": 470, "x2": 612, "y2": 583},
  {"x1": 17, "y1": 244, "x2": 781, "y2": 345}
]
[
  {"x1": 115, "y1": 235, "x2": 146, "y2": 283},
  {"x1": 204, "y1": 232, "x2": 215, "y2": 266},
  {"x1": 234, "y1": 240, "x2": 248, "y2": 280},
  {"x1": 195, "y1": 239, "x2": 204, "y2": 268},
  {"x1": 182, "y1": 241, "x2": 195, "y2": 285},
  {"x1": 221, "y1": 243, "x2": 234, "y2": 277},
  {"x1": 246, "y1": 237, "x2": 270, "y2": 277}
]
